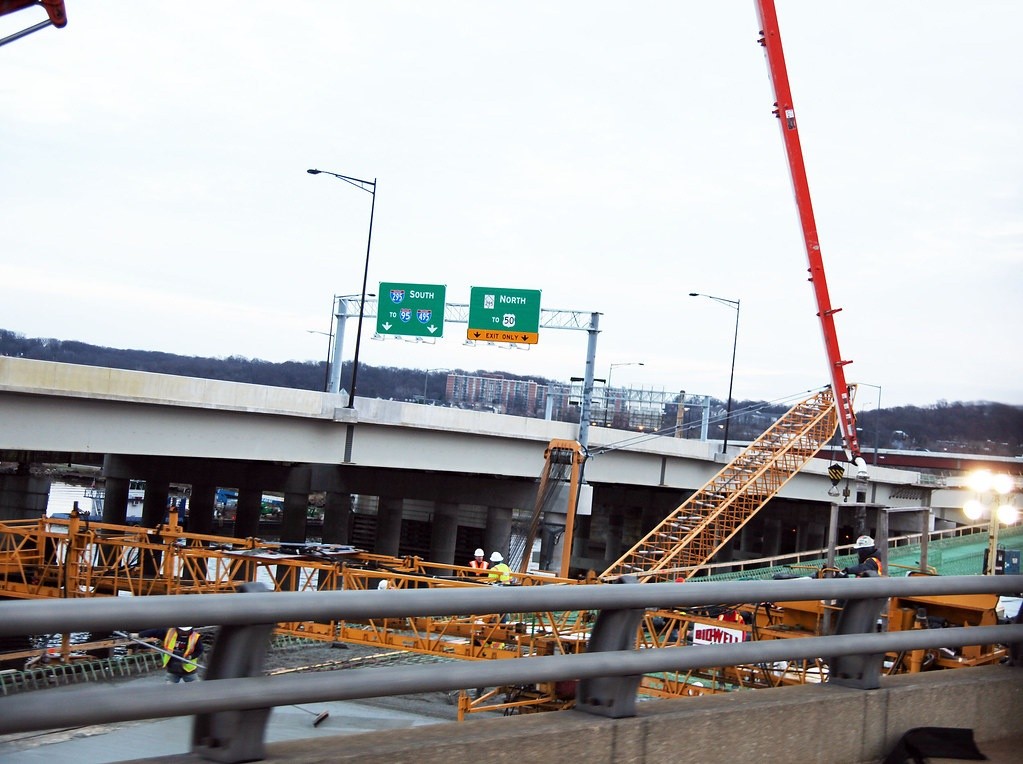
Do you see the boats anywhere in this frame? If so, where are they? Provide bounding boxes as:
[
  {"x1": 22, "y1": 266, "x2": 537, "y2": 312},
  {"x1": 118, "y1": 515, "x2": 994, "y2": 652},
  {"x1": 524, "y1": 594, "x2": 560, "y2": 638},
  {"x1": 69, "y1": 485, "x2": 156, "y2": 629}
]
[{"x1": 47, "y1": 473, "x2": 189, "y2": 536}]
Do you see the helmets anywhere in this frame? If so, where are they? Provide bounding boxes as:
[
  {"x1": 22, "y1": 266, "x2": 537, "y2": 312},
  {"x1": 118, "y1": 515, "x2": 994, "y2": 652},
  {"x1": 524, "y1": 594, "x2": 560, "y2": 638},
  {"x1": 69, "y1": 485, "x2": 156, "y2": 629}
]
[
  {"x1": 178, "y1": 626, "x2": 193, "y2": 631},
  {"x1": 853, "y1": 535, "x2": 875, "y2": 549},
  {"x1": 378, "y1": 580, "x2": 388, "y2": 591},
  {"x1": 473, "y1": 548, "x2": 484, "y2": 557},
  {"x1": 490, "y1": 552, "x2": 503, "y2": 561}
]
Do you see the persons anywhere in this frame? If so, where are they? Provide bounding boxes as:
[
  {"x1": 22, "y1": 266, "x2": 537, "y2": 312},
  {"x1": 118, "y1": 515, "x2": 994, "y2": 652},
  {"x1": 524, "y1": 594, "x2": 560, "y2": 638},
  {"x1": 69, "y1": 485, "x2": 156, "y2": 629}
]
[
  {"x1": 466, "y1": 548, "x2": 491, "y2": 578},
  {"x1": 488, "y1": 551, "x2": 513, "y2": 629},
  {"x1": 128, "y1": 627, "x2": 205, "y2": 684},
  {"x1": 842, "y1": 535, "x2": 882, "y2": 578}
]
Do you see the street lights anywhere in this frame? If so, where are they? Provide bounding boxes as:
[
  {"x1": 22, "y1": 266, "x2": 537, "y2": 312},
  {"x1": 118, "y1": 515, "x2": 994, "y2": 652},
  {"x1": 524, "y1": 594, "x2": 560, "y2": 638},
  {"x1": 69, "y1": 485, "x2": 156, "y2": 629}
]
[
  {"x1": 324, "y1": 293, "x2": 375, "y2": 393},
  {"x1": 307, "y1": 168, "x2": 377, "y2": 408},
  {"x1": 690, "y1": 292, "x2": 740, "y2": 454},
  {"x1": 965, "y1": 469, "x2": 1016, "y2": 575},
  {"x1": 308, "y1": 331, "x2": 335, "y2": 380},
  {"x1": 604, "y1": 362, "x2": 644, "y2": 427}
]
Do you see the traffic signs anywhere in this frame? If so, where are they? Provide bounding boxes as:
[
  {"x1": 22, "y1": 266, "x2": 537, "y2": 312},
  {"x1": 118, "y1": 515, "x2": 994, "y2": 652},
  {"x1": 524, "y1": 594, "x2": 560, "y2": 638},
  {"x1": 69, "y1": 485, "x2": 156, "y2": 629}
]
[
  {"x1": 466, "y1": 286, "x2": 541, "y2": 344},
  {"x1": 377, "y1": 281, "x2": 446, "y2": 338}
]
